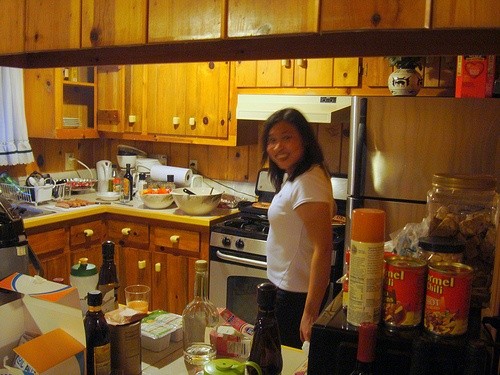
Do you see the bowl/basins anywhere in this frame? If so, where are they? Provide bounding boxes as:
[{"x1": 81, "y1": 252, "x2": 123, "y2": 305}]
[
  {"x1": 170, "y1": 187, "x2": 224, "y2": 215},
  {"x1": 139, "y1": 194, "x2": 174, "y2": 208}
]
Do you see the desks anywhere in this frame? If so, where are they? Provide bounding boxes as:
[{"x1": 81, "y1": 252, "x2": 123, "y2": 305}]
[{"x1": 83, "y1": 304, "x2": 309, "y2": 375}]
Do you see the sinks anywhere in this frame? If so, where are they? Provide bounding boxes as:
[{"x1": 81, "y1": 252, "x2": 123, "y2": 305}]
[{"x1": 10, "y1": 198, "x2": 56, "y2": 219}]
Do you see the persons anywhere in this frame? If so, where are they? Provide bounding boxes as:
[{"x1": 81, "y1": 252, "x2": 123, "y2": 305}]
[{"x1": 260, "y1": 109, "x2": 337, "y2": 349}]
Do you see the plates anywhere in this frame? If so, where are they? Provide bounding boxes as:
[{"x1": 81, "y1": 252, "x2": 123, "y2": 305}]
[
  {"x1": 63, "y1": 118, "x2": 80, "y2": 129},
  {"x1": 96, "y1": 192, "x2": 120, "y2": 201},
  {"x1": 26, "y1": 170, "x2": 43, "y2": 197}
]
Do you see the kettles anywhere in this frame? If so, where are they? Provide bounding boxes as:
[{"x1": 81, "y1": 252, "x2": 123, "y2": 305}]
[{"x1": 95, "y1": 160, "x2": 112, "y2": 191}]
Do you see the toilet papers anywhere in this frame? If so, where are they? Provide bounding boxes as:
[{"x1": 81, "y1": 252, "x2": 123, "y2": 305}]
[{"x1": 150, "y1": 164, "x2": 192, "y2": 186}]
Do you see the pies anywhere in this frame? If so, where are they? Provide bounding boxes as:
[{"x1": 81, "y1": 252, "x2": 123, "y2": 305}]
[{"x1": 251, "y1": 201, "x2": 270, "y2": 209}]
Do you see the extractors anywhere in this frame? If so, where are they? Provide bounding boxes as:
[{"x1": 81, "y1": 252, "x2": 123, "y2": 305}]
[{"x1": 236, "y1": 94, "x2": 353, "y2": 124}]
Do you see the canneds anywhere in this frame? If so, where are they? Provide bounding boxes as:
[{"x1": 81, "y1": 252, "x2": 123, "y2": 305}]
[
  {"x1": 382, "y1": 258, "x2": 474, "y2": 336},
  {"x1": 105, "y1": 307, "x2": 142, "y2": 375}
]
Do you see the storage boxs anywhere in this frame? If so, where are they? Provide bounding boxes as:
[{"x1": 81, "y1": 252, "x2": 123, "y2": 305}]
[
  {"x1": 0, "y1": 271, "x2": 88, "y2": 375},
  {"x1": 455, "y1": 55, "x2": 496, "y2": 99}
]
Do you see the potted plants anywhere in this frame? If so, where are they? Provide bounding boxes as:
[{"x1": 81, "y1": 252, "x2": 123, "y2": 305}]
[{"x1": 384, "y1": 56, "x2": 423, "y2": 97}]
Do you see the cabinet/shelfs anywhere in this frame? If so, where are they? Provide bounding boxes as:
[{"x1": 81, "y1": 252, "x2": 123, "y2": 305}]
[
  {"x1": 0, "y1": 0, "x2": 500, "y2": 148},
  {"x1": 22, "y1": 212, "x2": 212, "y2": 316}
]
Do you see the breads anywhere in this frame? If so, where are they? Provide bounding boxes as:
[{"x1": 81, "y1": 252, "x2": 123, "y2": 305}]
[{"x1": 55, "y1": 199, "x2": 89, "y2": 208}]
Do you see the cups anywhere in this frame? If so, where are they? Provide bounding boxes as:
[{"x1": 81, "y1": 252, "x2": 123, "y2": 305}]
[
  {"x1": 119, "y1": 180, "x2": 130, "y2": 202},
  {"x1": 124, "y1": 284, "x2": 152, "y2": 314}
]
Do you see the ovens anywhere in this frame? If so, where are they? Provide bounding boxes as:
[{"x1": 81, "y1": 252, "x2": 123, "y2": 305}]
[{"x1": 208, "y1": 249, "x2": 334, "y2": 324}]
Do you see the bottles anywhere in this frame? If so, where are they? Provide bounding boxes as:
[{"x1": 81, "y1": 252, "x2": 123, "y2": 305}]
[
  {"x1": 97, "y1": 241, "x2": 119, "y2": 311},
  {"x1": 69, "y1": 257, "x2": 99, "y2": 315},
  {"x1": 63, "y1": 68, "x2": 70, "y2": 81},
  {"x1": 82, "y1": 291, "x2": 113, "y2": 375},
  {"x1": 123, "y1": 163, "x2": 134, "y2": 200},
  {"x1": 202, "y1": 359, "x2": 264, "y2": 375},
  {"x1": 165, "y1": 175, "x2": 176, "y2": 189},
  {"x1": 182, "y1": 260, "x2": 219, "y2": 365},
  {"x1": 137, "y1": 172, "x2": 148, "y2": 195}
]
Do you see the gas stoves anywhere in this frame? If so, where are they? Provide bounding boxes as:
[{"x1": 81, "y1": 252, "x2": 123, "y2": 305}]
[{"x1": 209, "y1": 170, "x2": 348, "y2": 258}]
